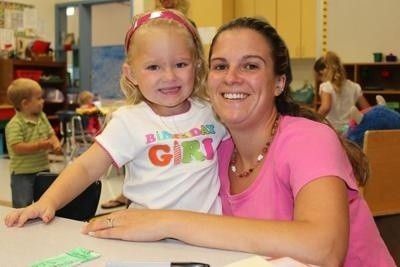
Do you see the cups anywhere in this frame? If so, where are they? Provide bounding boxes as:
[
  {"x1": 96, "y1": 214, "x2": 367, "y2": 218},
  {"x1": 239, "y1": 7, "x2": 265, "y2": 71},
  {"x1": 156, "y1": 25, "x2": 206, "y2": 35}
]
[{"x1": 374, "y1": 52, "x2": 382, "y2": 62}]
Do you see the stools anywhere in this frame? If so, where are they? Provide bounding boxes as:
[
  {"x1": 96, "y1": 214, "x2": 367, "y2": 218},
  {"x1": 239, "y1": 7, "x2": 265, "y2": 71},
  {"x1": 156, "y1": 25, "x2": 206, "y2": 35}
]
[{"x1": 55, "y1": 110, "x2": 87, "y2": 157}]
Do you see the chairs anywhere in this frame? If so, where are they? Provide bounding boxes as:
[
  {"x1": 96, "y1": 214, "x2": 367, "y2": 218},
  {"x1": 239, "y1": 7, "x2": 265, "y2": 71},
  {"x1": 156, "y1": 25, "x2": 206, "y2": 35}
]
[
  {"x1": 33, "y1": 171, "x2": 101, "y2": 222},
  {"x1": 357, "y1": 129, "x2": 400, "y2": 217}
]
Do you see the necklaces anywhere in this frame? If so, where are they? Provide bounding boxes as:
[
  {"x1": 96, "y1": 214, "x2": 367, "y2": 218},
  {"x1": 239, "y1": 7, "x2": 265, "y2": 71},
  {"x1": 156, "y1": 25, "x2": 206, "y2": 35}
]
[{"x1": 231, "y1": 113, "x2": 281, "y2": 180}]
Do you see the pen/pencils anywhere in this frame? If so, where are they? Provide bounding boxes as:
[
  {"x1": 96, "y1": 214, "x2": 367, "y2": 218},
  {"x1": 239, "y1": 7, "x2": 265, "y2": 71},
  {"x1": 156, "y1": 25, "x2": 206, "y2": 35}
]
[{"x1": 106, "y1": 261, "x2": 210, "y2": 267}]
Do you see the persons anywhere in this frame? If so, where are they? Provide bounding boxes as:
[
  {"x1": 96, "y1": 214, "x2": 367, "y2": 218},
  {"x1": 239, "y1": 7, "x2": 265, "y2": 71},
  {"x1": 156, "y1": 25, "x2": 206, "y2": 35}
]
[
  {"x1": 78, "y1": 91, "x2": 102, "y2": 140},
  {"x1": 4, "y1": 77, "x2": 60, "y2": 208},
  {"x1": 3, "y1": 6, "x2": 230, "y2": 229},
  {"x1": 84, "y1": 14, "x2": 398, "y2": 267},
  {"x1": 317, "y1": 50, "x2": 371, "y2": 131},
  {"x1": 314, "y1": 59, "x2": 326, "y2": 81}
]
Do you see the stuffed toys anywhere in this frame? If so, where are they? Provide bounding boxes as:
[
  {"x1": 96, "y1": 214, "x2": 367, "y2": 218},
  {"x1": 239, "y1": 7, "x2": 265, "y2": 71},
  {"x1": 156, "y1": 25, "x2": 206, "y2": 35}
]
[{"x1": 345, "y1": 95, "x2": 400, "y2": 150}]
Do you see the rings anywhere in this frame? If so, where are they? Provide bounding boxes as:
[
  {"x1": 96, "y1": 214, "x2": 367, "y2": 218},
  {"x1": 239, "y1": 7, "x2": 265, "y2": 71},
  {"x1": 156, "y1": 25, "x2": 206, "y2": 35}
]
[{"x1": 107, "y1": 217, "x2": 113, "y2": 228}]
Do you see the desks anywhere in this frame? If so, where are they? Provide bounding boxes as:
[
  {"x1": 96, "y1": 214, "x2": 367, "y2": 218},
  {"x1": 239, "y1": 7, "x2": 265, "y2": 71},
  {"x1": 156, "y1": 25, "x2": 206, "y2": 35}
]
[{"x1": 0, "y1": 206, "x2": 310, "y2": 267}]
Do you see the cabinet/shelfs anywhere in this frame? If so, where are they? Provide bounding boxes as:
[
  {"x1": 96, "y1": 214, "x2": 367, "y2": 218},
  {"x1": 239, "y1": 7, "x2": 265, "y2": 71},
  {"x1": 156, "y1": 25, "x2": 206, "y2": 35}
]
[
  {"x1": 313, "y1": 61, "x2": 400, "y2": 113},
  {"x1": 0, "y1": 60, "x2": 66, "y2": 153}
]
